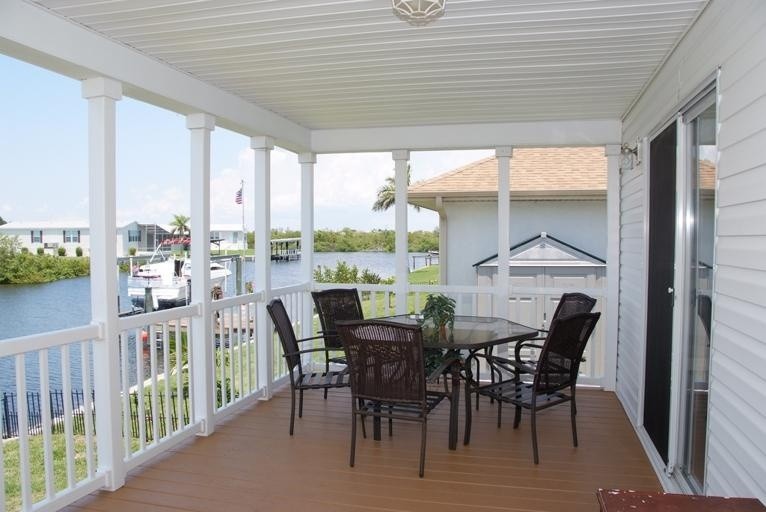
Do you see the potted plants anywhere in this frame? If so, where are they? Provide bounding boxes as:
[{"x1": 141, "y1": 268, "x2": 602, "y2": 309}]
[{"x1": 411, "y1": 291, "x2": 456, "y2": 339}]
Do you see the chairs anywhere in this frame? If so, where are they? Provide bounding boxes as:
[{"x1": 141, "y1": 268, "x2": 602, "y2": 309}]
[
  {"x1": 463, "y1": 311, "x2": 602, "y2": 465},
  {"x1": 266, "y1": 297, "x2": 367, "y2": 439},
  {"x1": 311, "y1": 288, "x2": 365, "y2": 399},
  {"x1": 489, "y1": 292, "x2": 597, "y2": 415}
]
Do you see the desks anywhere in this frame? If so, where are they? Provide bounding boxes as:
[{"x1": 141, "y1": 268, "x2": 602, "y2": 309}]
[
  {"x1": 597, "y1": 487, "x2": 766, "y2": 512},
  {"x1": 349, "y1": 313, "x2": 539, "y2": 451},
  {"x1": 328, "y1": 319, "x2": 461, "y2": 478}
]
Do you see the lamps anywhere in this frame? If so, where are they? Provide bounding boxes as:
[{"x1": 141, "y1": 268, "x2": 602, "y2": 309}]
[
  {"x1": 620, "y1": 142, "x2": 641, "y2": 170},
  {"x1": 390, "y1": 0, "x2": 447, "y2": 27}
]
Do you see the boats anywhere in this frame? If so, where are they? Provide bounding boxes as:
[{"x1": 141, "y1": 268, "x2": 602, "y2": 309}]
[{"x1": 125, "y1": 237, "x2": 232, "y2": 310}]
